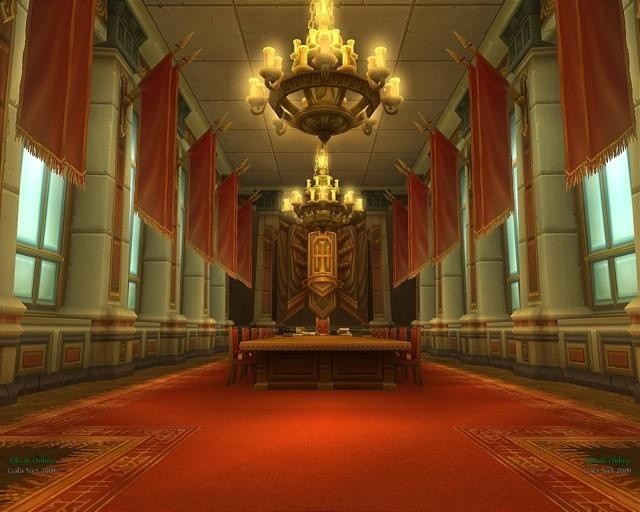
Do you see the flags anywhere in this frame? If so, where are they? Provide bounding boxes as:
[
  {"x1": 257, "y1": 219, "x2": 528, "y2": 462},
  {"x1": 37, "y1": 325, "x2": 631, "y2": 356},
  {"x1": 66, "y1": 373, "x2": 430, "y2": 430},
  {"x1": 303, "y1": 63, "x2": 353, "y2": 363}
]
[
  {"x1": 466, "y1": 52, "x2": 512, "y2": 238},
  {"x1": 188, "y1": 127, "x2": 217, "y2": 264},
  {"x1": 430, "y1": 125, "x2": 462, "y2": 260},
  {"x1": 215, "y1": 171, "x2": 239, "y2": 272},
  {"x1": 406, "y1": 170, "x2": 429, "y2": 278},
  {"x1": 139, "y1": 52, "x2": 180, "y2": 239},
  {"x1": 553, "y1": 1, "x2": 633, "y2": 189},
  {"x1": 235, "y1": 200, "x2": 254, "y2": 289},
  {"x1": 393, "y1": 195, "x2": 410, "y2": 287},
  {"x1": 18, "y1": 1, "x2": 97, "y2": 183}
]
